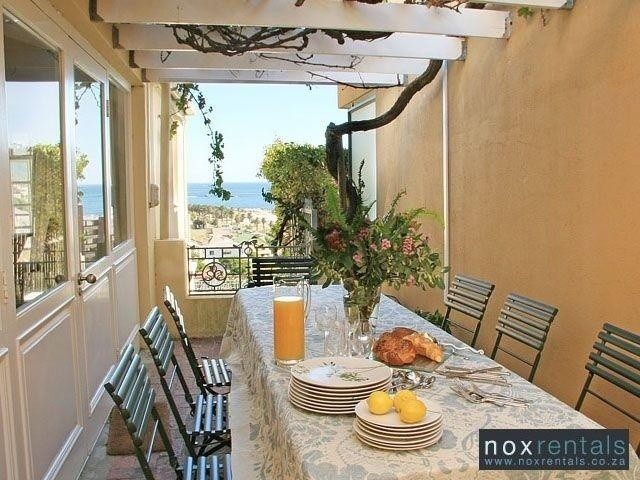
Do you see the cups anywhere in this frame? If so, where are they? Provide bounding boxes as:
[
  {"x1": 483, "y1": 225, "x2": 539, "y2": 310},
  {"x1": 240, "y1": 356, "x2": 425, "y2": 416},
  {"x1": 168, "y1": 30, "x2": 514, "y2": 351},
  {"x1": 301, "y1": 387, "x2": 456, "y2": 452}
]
[
  {"x1": 273, "y1": 275, "x2": 312, "y2": 367},
  {"x1": 315, "y1": 305, "x2": 374, "y2": 359}
]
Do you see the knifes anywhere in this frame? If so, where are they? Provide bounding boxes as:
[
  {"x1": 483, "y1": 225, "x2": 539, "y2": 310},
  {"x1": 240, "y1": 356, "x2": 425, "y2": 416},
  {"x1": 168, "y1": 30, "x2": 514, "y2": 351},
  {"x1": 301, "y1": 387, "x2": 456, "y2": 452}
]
[{"x1": 436, "y1": 364, "x2": 513, "y2": 387}]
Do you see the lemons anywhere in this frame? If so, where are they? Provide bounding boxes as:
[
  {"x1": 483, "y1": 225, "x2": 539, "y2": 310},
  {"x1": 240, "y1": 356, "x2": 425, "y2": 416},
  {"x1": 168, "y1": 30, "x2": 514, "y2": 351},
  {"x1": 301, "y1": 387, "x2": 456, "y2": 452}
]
[
  {"x1": 400, "y1": 398, "x2": 427, "y2": 423},
  {"x1": 366, "y1": 392, "x2": 394, "y2": 415},
  {"x1": 393, "y1": 390, "x2": 414, "y2": 413}
]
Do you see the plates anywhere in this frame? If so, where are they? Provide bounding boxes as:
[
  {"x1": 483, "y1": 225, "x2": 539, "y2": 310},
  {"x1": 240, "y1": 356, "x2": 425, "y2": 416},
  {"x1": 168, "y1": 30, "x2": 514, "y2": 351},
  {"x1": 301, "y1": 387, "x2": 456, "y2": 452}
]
[{"x1": 288, "y1": 357, "x2": 445, "y2": 451}]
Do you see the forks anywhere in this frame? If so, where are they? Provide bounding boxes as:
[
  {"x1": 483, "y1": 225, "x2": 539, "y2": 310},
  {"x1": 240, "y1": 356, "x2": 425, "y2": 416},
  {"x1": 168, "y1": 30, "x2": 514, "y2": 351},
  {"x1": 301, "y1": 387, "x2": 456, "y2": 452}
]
[{"x1": 450, "y1": 383, "x2": 533, "y2": 409}]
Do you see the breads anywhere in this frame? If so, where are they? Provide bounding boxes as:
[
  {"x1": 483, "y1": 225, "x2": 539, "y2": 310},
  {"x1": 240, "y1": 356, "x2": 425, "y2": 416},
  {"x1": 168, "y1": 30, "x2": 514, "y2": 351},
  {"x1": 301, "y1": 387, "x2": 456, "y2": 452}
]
[
  {"x1": 393, "y1": 327, "x2": 443, "y2": 363},
  {"x1": 373, "y1": 333, "x2": 416, "y2": 366}
]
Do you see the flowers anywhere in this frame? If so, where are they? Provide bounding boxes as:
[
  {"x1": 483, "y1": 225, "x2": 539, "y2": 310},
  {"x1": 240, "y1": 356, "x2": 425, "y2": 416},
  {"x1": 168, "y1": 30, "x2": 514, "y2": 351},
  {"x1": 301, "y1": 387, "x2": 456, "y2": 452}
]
[{"x1": 256, "y1": 137, "x2": 452, "y2": 337}]
